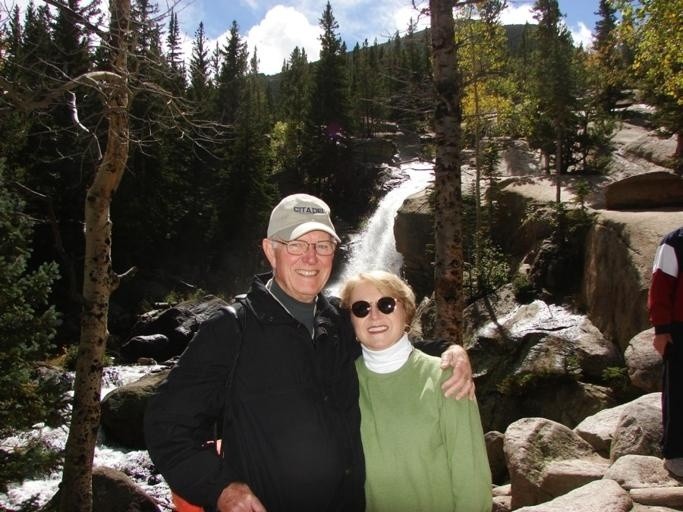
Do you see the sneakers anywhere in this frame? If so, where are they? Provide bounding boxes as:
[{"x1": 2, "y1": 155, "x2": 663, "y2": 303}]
[{"x1": 663, "y1": 458, "x2": 683, "y2": 477}]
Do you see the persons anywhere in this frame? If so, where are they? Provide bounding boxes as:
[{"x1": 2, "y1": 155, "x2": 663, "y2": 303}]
[
  {"x1": 332, "y1": 271, "x2": 494, "y2": 511},
  {"x1": 648, "y1": 227, "x2": 682, "y2": 481},
  {"x1": 141, "y1": 191, "x2": 478, "y2": 512}
]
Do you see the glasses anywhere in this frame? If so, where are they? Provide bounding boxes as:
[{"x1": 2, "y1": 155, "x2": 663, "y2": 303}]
[
  {"x1": 348, "y1": 296, "x2": 402, "y2": 318},
  {"x1": 273, "y1": 240, "x2": 337, "y2": 256}
]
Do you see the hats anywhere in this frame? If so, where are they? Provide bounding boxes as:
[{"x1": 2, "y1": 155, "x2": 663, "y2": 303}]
[{"x1": 266, "y1": 193, "x2": 342, "y2": 243}]
